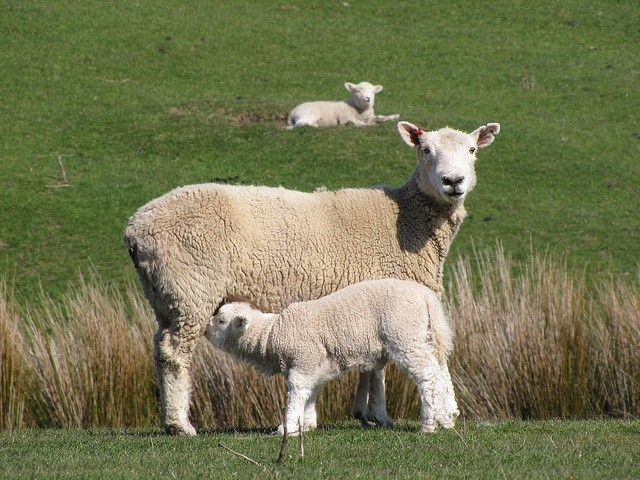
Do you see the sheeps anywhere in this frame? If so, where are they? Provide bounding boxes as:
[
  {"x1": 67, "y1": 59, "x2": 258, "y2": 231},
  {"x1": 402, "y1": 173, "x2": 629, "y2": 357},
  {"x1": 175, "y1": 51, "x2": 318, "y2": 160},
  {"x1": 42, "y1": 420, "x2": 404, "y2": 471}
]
[
  {"x1": 127, "y1": 121, "x2": 504, "y2": 436},
  {"x1": 208, "y1": 280, "x2": 462, "y2": 437},
  {"x1": 286, "y1": 80, "x2": 401, "y2": 131}
]
